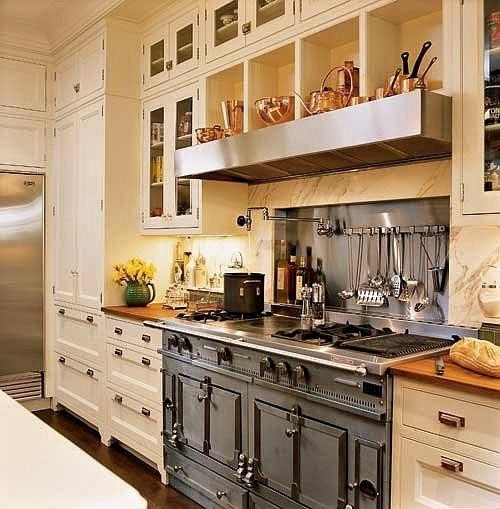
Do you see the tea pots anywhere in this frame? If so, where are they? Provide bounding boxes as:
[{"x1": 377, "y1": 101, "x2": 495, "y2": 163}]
[
  {"x1": 294, "y1": 63, "x2": 354, "y2": 115},
  {"x1": 125, "y1": 279, "x2": 157, "y2": 307}
]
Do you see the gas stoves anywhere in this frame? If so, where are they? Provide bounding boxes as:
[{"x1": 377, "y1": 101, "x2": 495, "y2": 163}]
[{"x1": 144, "y1": 302, "x2": 456, "y2": 419}]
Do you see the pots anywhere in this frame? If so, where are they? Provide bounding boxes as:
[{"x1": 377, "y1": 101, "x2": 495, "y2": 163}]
[{"x1": 219, "y1": 268, "x2": 266, "y2": 316}]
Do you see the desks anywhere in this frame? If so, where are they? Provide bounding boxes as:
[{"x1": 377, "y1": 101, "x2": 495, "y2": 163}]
[{"x1": 0, "y1": 391, "x2": 150, "y2": 509}]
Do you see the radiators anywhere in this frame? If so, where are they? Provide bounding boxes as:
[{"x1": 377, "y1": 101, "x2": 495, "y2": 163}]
[{"x1": 0, "y1": 370, "x2": 44, "y2": 403}]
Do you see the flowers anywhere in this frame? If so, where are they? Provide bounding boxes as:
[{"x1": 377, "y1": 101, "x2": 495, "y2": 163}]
[{"x1": 111, "y1": 257, "x2": 157, "y2": 288}]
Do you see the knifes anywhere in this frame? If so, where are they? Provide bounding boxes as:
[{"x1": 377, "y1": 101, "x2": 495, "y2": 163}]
[{"x1": 434, "y1": 355, "x2": 446, "y2": 377}]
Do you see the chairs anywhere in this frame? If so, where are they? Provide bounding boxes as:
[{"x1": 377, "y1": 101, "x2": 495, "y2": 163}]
[{"x1": 139, "y1": 1, "x2": 201, "y2": 100}]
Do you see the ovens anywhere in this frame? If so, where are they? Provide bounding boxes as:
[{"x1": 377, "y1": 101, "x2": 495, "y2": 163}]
[{"x1": 160, "y1": 350, "x2": 391, "y2": 508}]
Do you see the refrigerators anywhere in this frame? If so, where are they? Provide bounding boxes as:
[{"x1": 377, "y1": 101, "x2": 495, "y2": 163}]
[{"x1": 1, "y1": 170, "x2": 46, "y2": 403}]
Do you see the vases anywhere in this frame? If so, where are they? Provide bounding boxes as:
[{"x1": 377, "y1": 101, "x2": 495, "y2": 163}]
[{"x1": 124, "y1": 281, "x2": 155, "y2": 307}]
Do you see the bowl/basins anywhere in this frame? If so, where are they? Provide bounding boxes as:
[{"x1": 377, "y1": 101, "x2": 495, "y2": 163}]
[{"x1": 190, "y1": 90, "x2": 295, "y2": 144}]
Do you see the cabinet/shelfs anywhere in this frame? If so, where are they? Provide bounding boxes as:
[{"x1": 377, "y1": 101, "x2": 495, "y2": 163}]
[
  {"x1": 390, "y1": 374, "x2": 500, "y2": 509},
  {"x1": 0, "y1": 105, "x2": 48, "y2": 176},
  {"x1": 0, "y1": 43, "x2": 47, "y2": 112},
  {"x1": 196, "y1": 1, "x2": 450, "y2": 145},
  {"x1": 300, "y1": 1, "x2": 351, "y2": 22},
  {"x1": 49, "y1": 302, "x2": 168, "y2": 487},
  {"x1": 450, "y1": 1, "x2": 500, "y2": 215},
  {"x1": 43, "y1": 17, "x2": 142, "y2": 118},
  {"x1": 200, "y1": 1, "x2": 295, "y2": 64},
  {"x1": 139, "y1": 74, "x2": 250, "y2": 238},
  {"x1": 46, "y1": 95, "x2": 251, "y2": 315}
]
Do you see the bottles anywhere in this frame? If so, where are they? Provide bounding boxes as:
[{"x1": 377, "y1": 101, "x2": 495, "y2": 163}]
[
  {"x1": 273, "y1": 234, "x2": 331, "y2": 333},
  {"x1": 167, "y1": 238, "x2": 222, "y2": 289},
  {"x1": 177, "y1": 110, "x2": 194, "y2": 137}
]
